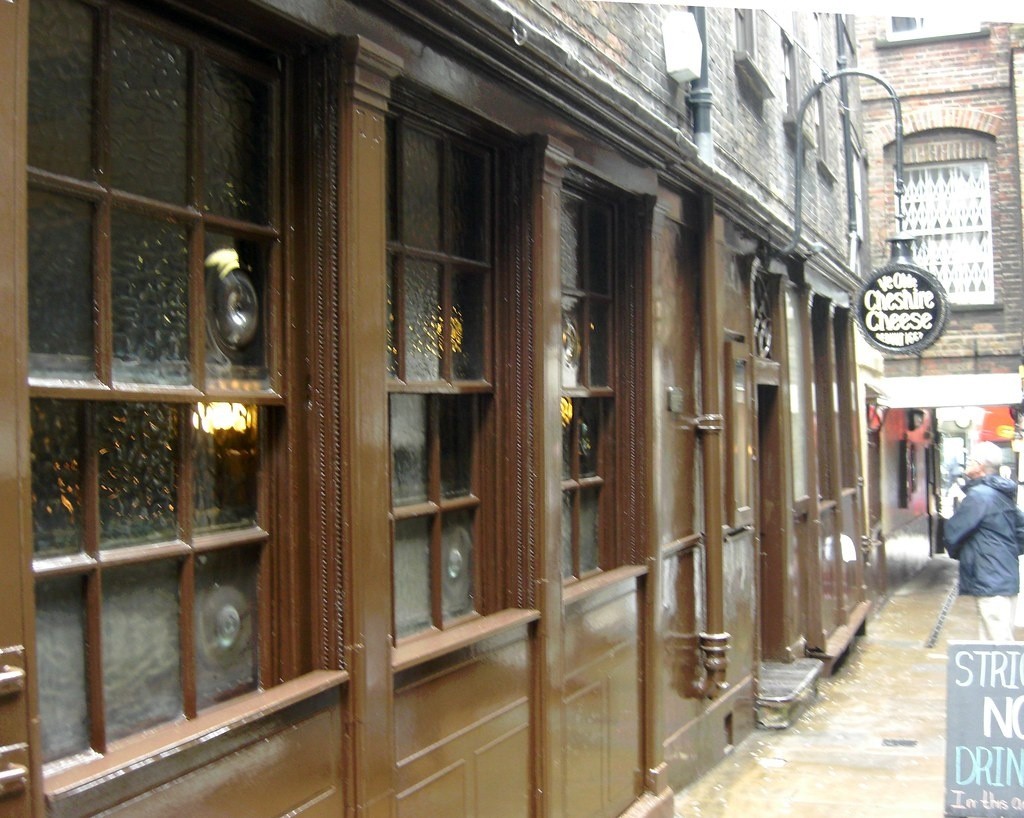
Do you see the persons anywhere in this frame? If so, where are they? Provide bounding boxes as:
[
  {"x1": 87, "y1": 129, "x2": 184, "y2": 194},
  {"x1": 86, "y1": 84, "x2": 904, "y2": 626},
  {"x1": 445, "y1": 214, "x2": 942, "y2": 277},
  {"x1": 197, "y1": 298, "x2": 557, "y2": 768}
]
[{"x1": 944, "y1": 440, "x2": 1024, "y2": 640}]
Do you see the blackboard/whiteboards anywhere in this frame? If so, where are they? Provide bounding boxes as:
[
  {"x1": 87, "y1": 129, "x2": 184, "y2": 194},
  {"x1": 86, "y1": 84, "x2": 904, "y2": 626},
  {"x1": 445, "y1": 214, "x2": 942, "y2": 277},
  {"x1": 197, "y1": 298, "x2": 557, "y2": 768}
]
[{"x1": 944, "y1": 640, "x2": 1024, "y2": 818}]
